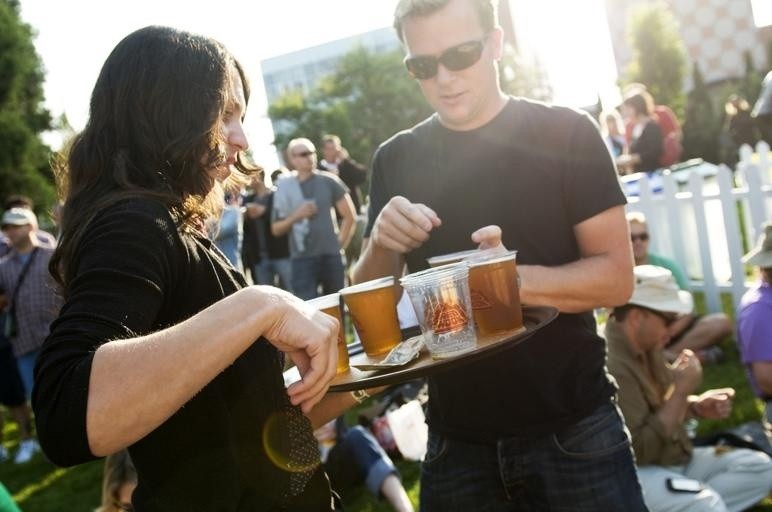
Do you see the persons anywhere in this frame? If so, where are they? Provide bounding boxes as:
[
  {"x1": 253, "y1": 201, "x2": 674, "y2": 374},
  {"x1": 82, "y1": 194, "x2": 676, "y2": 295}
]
[
  {"x1": 213, "y1": 132, "x2": 367, "y2": 301},
  {"x1": 314, "y1": 418, "x2": 416, "y2": 511},
  {"x1": 348, "y1": 1, "x2": 650, "y2": 512},
  {"x1": 93, "y1": 447, "x2": 138, "y2": 512},
  {"x1": 597, "y1": 210, "x2": 772, "y2": 512},
  {"x1": 1, "y1": 196, "x2": 63, "y2": 464},
  {"x1": 31, "y1": 25, "x2": 395, "y2": 512},
  {"x1": 598, "y1": 68, "x2": 772, "y2": 176}
]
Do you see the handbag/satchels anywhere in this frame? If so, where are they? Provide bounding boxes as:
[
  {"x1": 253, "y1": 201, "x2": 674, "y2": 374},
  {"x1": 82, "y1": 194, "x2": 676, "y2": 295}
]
[{"x1": 1, "y1": 311, "x2": 18, "y2": 340}]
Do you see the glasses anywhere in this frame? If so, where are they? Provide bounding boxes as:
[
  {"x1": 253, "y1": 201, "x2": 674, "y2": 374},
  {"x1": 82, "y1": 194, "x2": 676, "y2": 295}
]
[
  {"x1": 402, "y1": 28, "x2": 493, "y2": 81},
  {"x1": 636, "y1": 306, "x2": 678, "y2": 328},
  {"x1": 295, "y1": 149, "x2": 315, "y2": 159},
  {"x1": 632, "y1": 231, "x2": 652, "y2": 242}
]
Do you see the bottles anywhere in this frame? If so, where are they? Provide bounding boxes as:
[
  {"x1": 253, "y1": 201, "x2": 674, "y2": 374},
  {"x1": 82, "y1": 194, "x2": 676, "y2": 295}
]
[{"x1": 660, "y1": 350, "x2": 694, "y2": 402}]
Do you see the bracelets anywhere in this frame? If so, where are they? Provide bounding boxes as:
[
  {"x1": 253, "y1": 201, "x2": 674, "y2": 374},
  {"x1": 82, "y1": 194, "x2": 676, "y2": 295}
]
[{"x1": 350, "y1": 389, "x2": 371, "y2": 404}]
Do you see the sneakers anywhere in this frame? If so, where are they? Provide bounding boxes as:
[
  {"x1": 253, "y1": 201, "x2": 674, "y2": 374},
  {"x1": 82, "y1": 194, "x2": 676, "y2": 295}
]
[{"x1": 14, "y1": 438, "x2": 41, "y2": 465}]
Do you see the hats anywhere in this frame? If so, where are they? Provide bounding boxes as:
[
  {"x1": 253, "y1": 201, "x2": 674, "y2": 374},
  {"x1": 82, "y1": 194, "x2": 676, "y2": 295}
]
[
  {"x1": 2, "y1": 207, "x2": 39, "y2": 230},
  {"x1": 629, "y1": 264, "x2": 695, "y2": 315},
  {"x1": 739, "y1": 222, "x2": 772, "y2": 269}
]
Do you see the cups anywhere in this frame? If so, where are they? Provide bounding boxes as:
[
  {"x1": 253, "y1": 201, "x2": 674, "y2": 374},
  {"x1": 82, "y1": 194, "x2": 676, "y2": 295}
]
[
  {"x1": 404, "y1": 261, "x2": 474, "y2": 359},
  {"x1": 301, "y1": 291, "x2": 351, "y2": 377},
  {"x1": 338, "y1": 275, "x2": 403, "y2": 358},
  {"x1": 428, "y1": 247, "x2": 474, "y2": 266},
  {"x1": 466, "y1": 246, "x2": 525, "y2": 338}
]
